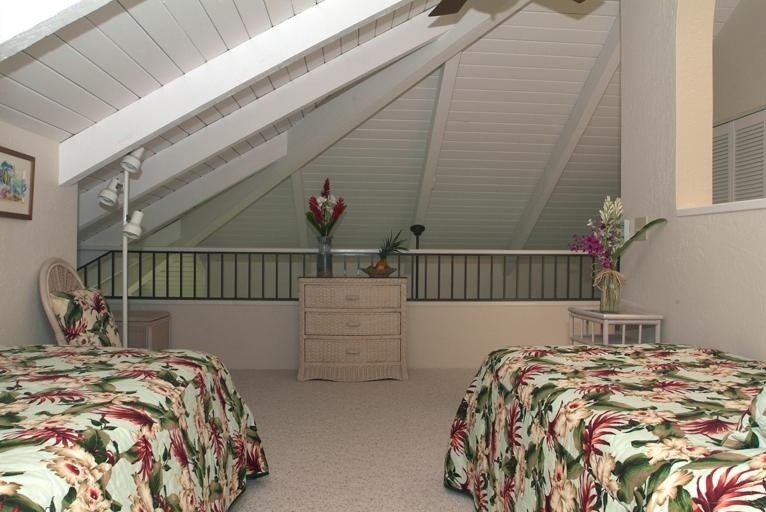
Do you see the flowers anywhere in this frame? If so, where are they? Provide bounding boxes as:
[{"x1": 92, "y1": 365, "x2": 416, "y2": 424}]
[
  {"x1": 304, "y1": 177, "x2": 347, "y2": 237},
  {"x1": 566, "y1": 193, "x2": 667, "y2": 269}
]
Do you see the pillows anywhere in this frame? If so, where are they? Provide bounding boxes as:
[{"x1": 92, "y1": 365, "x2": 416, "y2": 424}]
[{"x1": 49, "y1": 288, "x2": 122, "y2": 348}]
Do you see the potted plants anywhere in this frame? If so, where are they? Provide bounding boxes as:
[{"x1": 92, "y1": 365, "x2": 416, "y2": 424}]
[{"x1": 360, "y1": 228, "x2": 408, "y2": 277}]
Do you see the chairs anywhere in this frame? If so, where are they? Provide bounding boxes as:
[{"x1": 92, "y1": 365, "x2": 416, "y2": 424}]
[{"x1": 38, "y1": 257, "x2": 121, "y2": 348}]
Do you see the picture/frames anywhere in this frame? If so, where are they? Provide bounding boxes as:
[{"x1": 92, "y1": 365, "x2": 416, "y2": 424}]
[{"x1": 0, "y1": 147, "x2": 36, "y2": 221}]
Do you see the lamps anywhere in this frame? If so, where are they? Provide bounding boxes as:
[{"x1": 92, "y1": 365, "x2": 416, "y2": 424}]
[{"x1": 97, "y1": 147, "x2": 146, "y2": 352}]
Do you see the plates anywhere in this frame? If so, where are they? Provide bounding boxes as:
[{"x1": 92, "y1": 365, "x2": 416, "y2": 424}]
[{"x1": 360, "y1": 266, "x2": 398, "y2": 277}]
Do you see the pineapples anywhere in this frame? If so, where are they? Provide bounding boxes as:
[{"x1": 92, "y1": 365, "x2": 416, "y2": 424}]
[{"x1": 375, "y1": 229, "x2": 409, "y2": 271}]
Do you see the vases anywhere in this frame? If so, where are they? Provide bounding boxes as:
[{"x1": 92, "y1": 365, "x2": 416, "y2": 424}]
[
  {"x1": 598, "y1": 270, "x2": 622, "y2": 312},
  {"x1": 316, "y1": 235, "x2": 333, "y2": 279}
]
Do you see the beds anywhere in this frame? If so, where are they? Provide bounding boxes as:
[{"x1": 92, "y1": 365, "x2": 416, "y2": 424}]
[
  {"x1": 2, "y1": 342, "x2": 226, "y2": 512},
  {"x1": 485, "y1": 344, "x2": 765, "y2": 511}
]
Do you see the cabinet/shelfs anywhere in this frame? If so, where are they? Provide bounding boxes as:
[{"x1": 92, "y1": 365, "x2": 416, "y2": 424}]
[
  {"x1": 113, "y1": 309, "x2": 172, "y2": 352},
  {"x1": 299, "y1": 276, "x2": 409, "y2": 384}
]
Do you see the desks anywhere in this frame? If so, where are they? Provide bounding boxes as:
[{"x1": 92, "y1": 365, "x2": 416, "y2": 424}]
[{"x1": 570, "y1": 306, "x2": 664, "y2": 348}]
